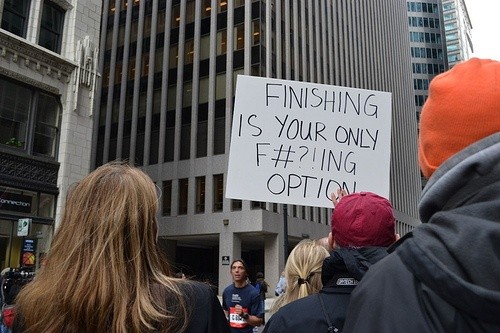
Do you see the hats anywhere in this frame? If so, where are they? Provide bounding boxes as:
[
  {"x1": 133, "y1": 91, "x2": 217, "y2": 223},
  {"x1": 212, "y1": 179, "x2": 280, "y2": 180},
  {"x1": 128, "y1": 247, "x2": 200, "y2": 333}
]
[
  {"x1": 418, "y1": 58, "x2": 500, "y2": 179},
  {"x1": 330, "y1": 192, "x2": 395, "y2": 247}
]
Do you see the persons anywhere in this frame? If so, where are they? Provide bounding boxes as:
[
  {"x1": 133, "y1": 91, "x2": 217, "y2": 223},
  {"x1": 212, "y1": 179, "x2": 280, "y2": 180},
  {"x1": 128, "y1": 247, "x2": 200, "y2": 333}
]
[
  {"x1": 343, "y1": 56, "x2": 500, "y2": 333},
  {"x1": 176, "y1": 236, "x2": 336, "y2": 333},
  {"x1": 12, "y1": 160, "x2": 231, "y2": 333},
  {"x1": 261, "y1": 188, "x2": 401, "y2": 333}
]
